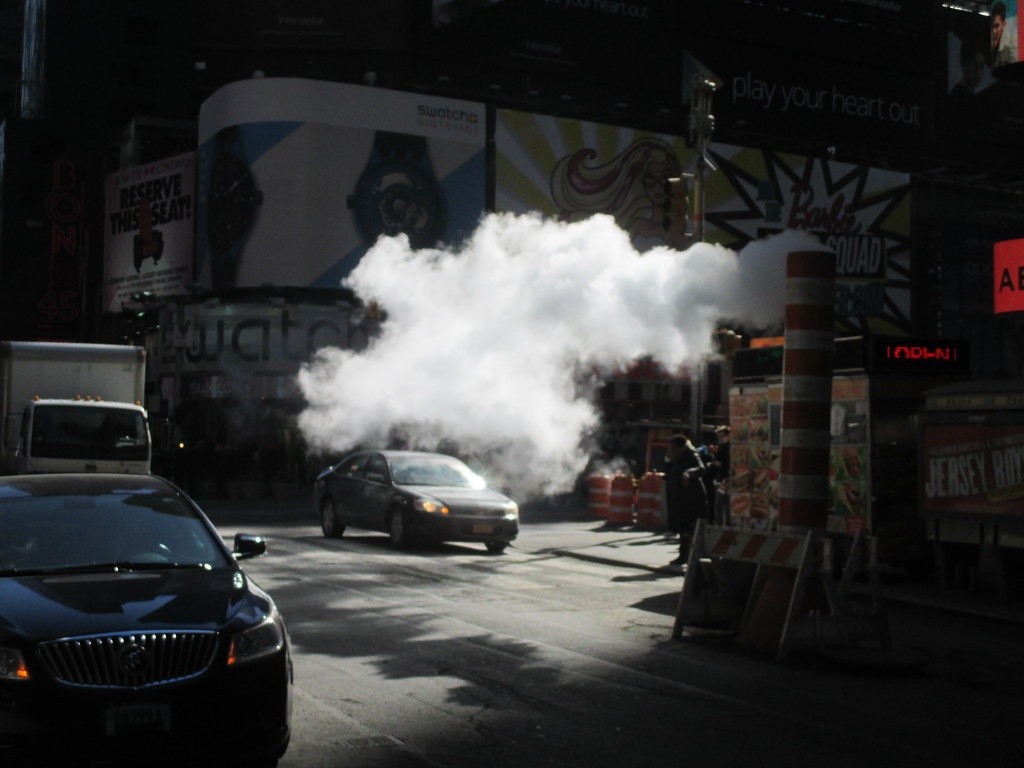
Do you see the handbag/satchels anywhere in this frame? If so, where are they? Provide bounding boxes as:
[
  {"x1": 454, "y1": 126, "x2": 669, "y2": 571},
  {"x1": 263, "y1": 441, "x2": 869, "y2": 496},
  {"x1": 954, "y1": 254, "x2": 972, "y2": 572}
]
[{"x1": 680, "y1": 471, "x2": 706, "y2": 505}]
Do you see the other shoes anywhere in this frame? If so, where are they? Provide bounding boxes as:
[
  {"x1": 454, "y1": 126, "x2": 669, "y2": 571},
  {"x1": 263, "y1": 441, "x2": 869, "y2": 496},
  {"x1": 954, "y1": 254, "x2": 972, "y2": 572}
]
[{"x1": 671, "y1": 556, "x2": 688, "y2": 566}]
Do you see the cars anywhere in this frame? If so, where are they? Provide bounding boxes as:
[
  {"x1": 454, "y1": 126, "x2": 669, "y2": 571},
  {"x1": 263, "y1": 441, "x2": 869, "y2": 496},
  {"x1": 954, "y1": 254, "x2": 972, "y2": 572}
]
[
  {"x1": 311, "y1": 450, "x2": 522, "y2": 552},
  {"x1": 0, "y1": 472, "x2": 296, "y2": 768}
]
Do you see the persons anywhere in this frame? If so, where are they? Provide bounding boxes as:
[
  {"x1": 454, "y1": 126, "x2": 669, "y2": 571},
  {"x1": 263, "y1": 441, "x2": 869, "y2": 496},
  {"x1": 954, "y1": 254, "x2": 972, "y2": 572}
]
[
  {"x1": 158, "y1": 433, "x2": 317, "y2": 502},
  {"x1": 663, "y1": 424, "x2": 730, "y2": 563}
]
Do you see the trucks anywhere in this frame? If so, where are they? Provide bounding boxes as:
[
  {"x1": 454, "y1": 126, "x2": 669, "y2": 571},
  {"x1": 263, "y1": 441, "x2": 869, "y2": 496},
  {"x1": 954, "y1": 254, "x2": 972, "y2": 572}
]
[{"x1": 1, "y1": 342, "x2": 154, "y2": 479}]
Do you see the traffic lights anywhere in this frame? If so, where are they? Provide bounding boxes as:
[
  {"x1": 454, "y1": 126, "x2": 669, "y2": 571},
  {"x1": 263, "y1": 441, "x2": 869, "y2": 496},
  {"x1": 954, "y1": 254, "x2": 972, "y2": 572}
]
[{"x1": 662, "y1": 175, "x2": 691, "y2": 234}]
